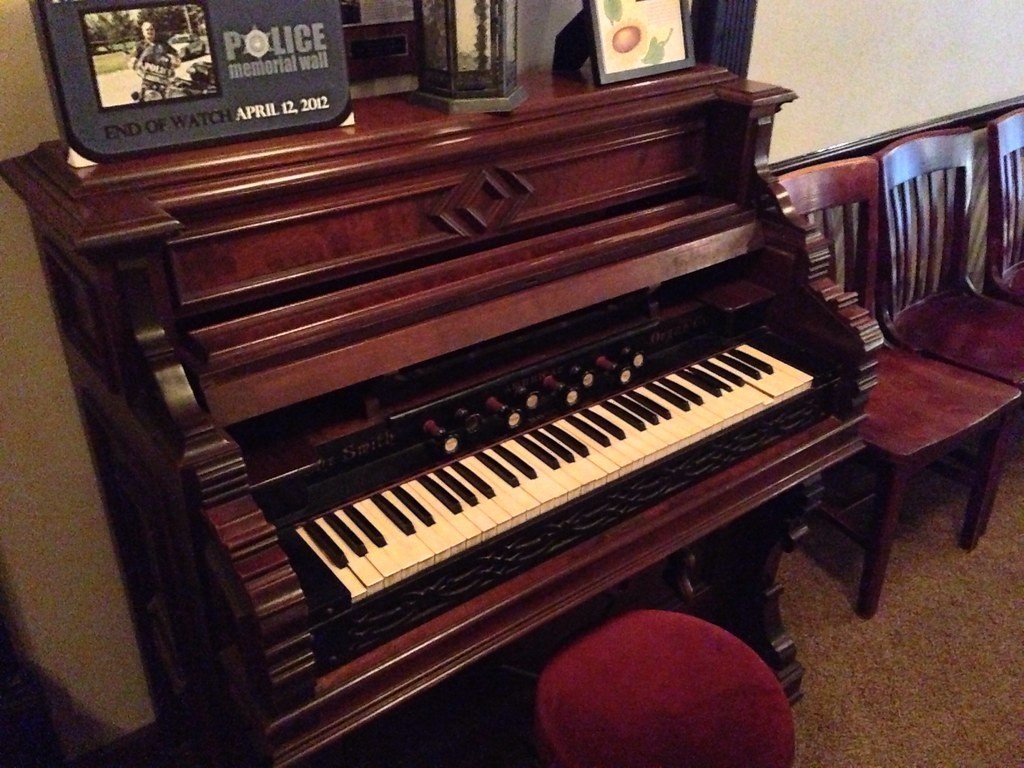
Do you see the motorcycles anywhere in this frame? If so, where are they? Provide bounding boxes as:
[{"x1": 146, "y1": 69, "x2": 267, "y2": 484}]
[{"x1": 128, "y1": 43, "x2": 175, "y2": 102}]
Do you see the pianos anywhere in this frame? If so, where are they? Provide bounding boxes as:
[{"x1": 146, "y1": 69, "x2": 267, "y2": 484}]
[{"x1": 2, "y1": 57, "x2": 887, "y2": 768}]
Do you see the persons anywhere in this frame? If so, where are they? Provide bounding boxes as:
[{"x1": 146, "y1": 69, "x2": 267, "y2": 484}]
[{"x1": 127, "y1": 22, "x2": 180, "y2": 103}]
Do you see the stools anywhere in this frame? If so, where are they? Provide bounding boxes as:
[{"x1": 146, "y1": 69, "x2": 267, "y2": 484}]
[{"x1": 534, "y1": 610, "x2": 795, "y2": 768}]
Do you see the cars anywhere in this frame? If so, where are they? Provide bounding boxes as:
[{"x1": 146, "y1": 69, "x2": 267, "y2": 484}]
[{"x1": 168, "y1": 33, "x2": 206, "y2": 60}]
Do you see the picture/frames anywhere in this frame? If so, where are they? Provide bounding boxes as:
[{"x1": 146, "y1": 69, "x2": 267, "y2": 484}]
[{"x1": 553, "y1": 0, "x2": 697, "y2": 87}]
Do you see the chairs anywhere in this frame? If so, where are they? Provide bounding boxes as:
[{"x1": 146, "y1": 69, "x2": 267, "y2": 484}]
[
  {"x1": 774, "y1": 158, "x2": 1022, "y2": 619},
  {"x1": 984, "y1": 107, "x2": 1024, "y2": 306},
  {"x1": 872, "y1": 127, "x2": 1024, "y2": 388}
]
